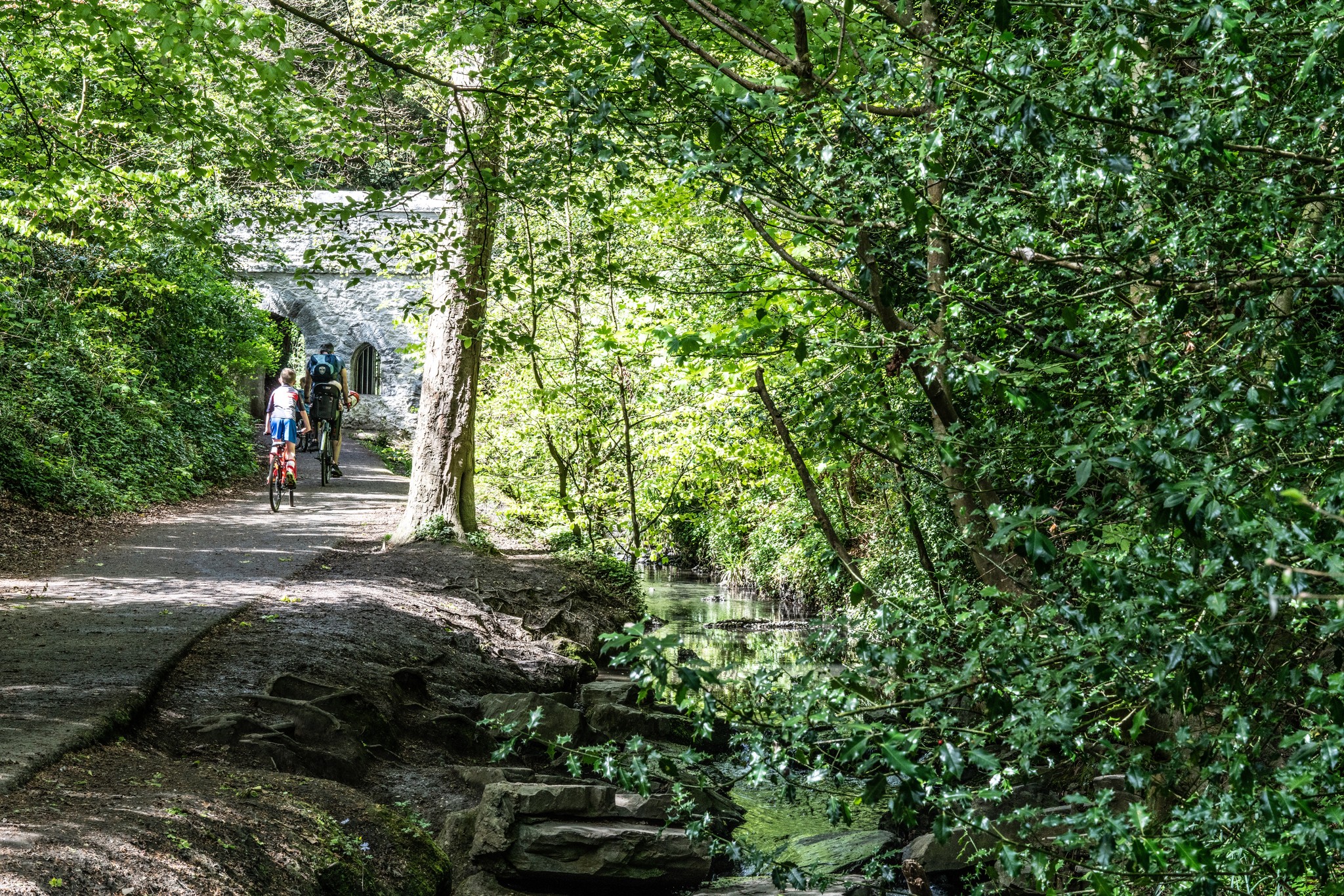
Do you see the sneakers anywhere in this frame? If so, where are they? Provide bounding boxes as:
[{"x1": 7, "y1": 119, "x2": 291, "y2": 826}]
[
  {"x1": 331, "y1": 463, "x2": 343, "y2": 477},
  {"x1": 306, "y1": 441, "x2": 319, "y2": 451},
  {"x1": 286, "y1": 470, "x2": 297, "y2": 489}
]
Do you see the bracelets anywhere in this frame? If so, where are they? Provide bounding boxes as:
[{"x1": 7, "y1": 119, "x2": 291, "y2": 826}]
[{"x1": 304, "y1": 396, "x2": 310, "y2": 400}]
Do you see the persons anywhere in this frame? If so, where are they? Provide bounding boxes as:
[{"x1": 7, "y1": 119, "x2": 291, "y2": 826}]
[
  {"x1": 294, "y1": 377, "x2": 311, "y2": 424},
  {"x1": 264, "y1": 369, "x2": 311, "y2": 489},
  {"x1": 304, "y1": 342, "x2": 353, "y2": 477}
]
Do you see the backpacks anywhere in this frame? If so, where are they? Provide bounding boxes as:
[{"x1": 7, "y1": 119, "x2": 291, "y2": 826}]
[{"x1": 310, "y1": 353, "x2": 338, "y2": 380}]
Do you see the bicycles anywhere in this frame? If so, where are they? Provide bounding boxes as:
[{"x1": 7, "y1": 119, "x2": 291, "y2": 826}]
[
  {"x1": 263, "y1": 429, "x2": 313, "y2": 512},
  {"x1": 282, "y1": 417, "x2": 314, "y2": 453},
  {"x1": 304, "y1": 399, "x2": 358, "y2": 487}
]
